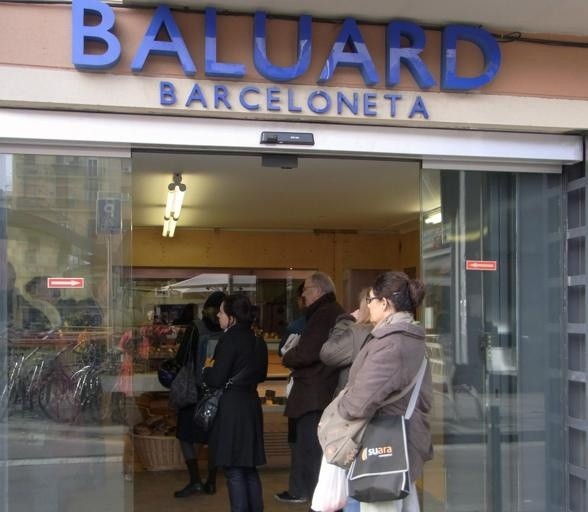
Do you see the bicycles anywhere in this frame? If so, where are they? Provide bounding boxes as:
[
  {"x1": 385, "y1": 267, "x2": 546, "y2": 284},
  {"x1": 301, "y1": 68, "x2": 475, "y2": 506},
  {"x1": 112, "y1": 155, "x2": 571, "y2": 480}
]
[{"x1": 1, "y1": 316, "x2": 126, "y2": 428}]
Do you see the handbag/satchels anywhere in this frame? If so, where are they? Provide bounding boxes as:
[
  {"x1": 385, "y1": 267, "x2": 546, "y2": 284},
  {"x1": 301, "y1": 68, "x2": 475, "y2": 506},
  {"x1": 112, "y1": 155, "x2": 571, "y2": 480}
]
[
  {"x1": 317, "y1": 385, "x2": 423, "y2": 502},
  {"x1": 157, "y1": 326, "x2": 223, "y2": 427}
]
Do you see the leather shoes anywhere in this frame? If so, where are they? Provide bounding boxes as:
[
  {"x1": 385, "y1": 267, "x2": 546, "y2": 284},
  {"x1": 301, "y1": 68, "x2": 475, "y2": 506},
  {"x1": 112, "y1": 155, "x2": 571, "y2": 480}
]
[{"x1": 174, "y1": 479, "x2": 215, "y2": 497}]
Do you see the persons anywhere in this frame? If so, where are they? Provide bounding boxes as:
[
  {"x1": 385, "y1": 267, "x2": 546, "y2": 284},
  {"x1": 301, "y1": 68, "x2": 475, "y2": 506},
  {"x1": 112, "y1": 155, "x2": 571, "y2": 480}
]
[
  {"x1": 8, "y1": 260, "x2": 105, "y2": 331},
  {"x1": 157, "y1": 291, "x2": 269, "y2": 512},
  {"x1": 273, "y1": 271, "x2": 434, "y2": 511}
]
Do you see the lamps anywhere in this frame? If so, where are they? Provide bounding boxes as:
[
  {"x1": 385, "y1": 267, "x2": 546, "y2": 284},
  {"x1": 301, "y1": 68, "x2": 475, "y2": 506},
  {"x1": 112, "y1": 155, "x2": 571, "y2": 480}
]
[{"x1": 162, "y1": 173, "x2": 186, "y2": 238}]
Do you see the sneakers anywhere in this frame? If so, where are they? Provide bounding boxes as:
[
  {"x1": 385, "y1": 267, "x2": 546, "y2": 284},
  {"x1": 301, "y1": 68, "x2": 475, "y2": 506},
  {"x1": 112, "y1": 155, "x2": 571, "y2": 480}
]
[{"x1": 274, "y1": 491, "x2": 306, "y2": 502}]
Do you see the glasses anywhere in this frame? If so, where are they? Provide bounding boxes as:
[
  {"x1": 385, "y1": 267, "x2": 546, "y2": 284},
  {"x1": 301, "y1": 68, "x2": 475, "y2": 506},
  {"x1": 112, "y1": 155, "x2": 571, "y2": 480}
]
[{"x1": 366, "y1": 297, "x2": 390, "y2": 305}]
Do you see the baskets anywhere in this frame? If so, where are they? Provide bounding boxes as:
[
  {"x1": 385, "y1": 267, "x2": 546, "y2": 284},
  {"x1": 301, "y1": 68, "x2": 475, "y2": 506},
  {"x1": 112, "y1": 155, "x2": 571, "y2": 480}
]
[{"x1": 133, "y1": 435, "x2": 202, "y2": 474}]
